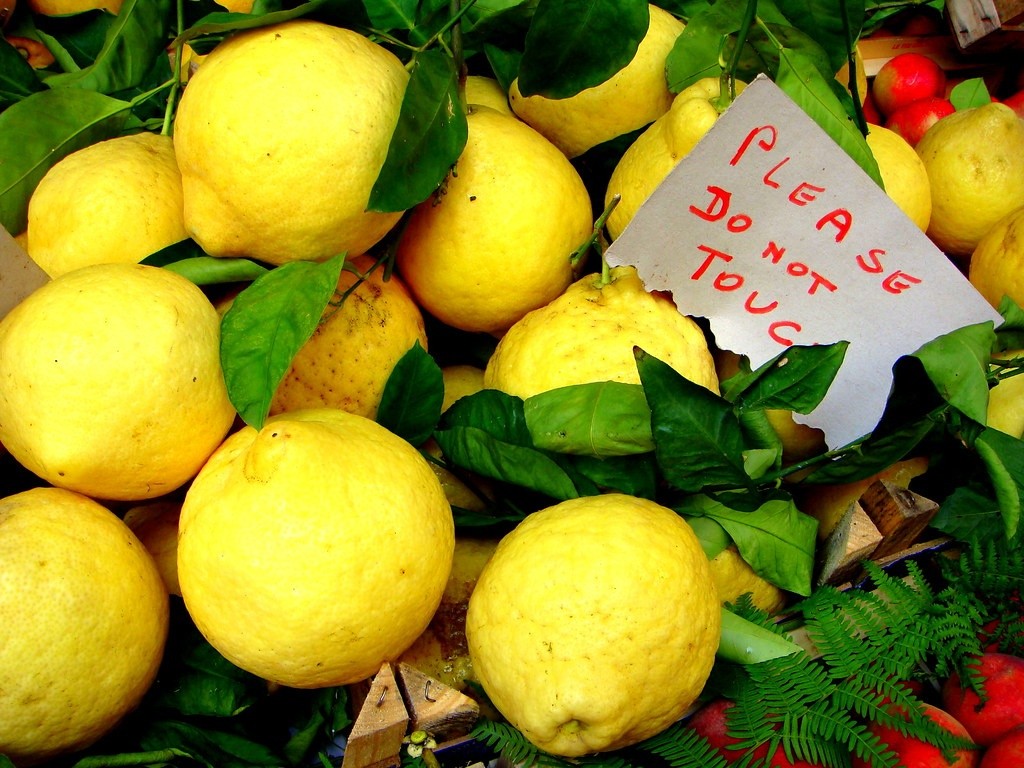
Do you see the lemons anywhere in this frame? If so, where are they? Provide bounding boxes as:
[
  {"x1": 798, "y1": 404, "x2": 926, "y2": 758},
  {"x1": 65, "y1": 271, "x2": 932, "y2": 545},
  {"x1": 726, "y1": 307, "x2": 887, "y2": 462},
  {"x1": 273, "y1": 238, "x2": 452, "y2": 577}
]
[{"x1": 0, "y1": 0, "x2": 1024, "y2": 757}]
